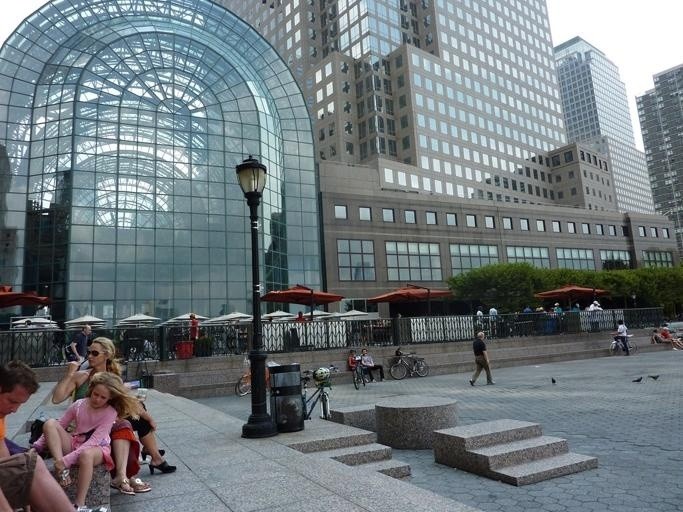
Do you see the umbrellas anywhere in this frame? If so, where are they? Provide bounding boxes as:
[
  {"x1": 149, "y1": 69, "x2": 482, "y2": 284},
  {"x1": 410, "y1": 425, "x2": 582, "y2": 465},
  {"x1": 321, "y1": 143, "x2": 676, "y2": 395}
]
[
  {"x1": 535, "y1": 284, "x2": 609, "y2": 310},
  {"x1": 370, "y1": 284, "x2": 452, "y2": 315}
]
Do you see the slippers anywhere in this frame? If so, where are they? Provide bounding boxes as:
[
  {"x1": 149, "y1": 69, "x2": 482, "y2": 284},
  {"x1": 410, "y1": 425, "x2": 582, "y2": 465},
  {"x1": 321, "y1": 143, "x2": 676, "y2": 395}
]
[
  {"x1": 129, "y1": 478, "x2": 151, "y2": 492},
  {"x1": 110, "y1": 478, "x2": 135, "y2": 495},
  {"x1": 55, "y1": 469, "x2": 72, "y2": 488}
]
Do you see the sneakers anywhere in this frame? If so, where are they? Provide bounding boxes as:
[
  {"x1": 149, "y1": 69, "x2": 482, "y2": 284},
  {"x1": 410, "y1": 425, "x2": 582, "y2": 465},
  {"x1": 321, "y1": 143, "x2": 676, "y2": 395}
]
[{"x1": 74, "y1": 504, "x2": 107, "y2": 512}]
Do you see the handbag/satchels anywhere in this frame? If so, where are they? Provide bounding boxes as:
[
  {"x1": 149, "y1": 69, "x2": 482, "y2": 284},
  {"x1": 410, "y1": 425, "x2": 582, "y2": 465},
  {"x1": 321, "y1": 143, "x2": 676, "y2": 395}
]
[{"x1": 29, "y1": 419, "x2": 53, "y2": 460}]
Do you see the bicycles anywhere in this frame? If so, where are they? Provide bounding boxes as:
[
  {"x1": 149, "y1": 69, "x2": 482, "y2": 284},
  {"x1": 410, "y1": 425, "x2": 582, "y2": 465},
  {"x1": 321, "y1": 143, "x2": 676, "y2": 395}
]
[
  {"x1": 301, "y1": 365, "x2": 338, "y2": 421},
  {"x1": 351, "y1": 352, "x2": 368, "y2": 389},
  {"x1": 390, "y1": 348, "x2": 430, "y2": 379},
  {"x1": 609, "y1": 333, "x2": 638, "y2": 357},
  {"x1": 235, "y1": 363, "x2": 280, "y2": 399}
]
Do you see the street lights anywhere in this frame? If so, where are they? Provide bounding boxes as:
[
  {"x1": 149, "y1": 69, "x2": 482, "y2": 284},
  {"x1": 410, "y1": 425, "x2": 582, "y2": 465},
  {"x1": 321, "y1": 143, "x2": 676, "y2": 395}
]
[
  {"x1": 234, "y1": 156, "x2": 280, "y2": 439},
  {"x1": 631, "y1": 290, "x2": 637, "y2": 308}
]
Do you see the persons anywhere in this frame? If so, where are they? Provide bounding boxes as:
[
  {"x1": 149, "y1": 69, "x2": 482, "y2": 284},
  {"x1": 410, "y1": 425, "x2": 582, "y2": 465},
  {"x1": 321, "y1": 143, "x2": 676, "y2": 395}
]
[
  {"x1": 70, "y1": 325, "x2": 90, "y2": 362},
  {"x1": 361, "y1": 349, "x2": 385, "y2": 383},
  {"x1": 348, "y1": 349, "x2": 368, "y2": 382},
  {"x1": 1, "y1": 359, "x2": 75, "y2": 512},
  {"x1": 614, "y1": 320, "x2": 629, "y2": 356},
  {"x1": 125, "y1": 402, "x2": 177, "y2": 474},
  {"x1": 53, "y1": 337, "x2": 152, "y2": 496},
  {"x1": 508, "y1": 301, "x2": 603, "y2": 337},
  {"x1": 489, "y1": 304, "x2": 498, "y2": 334},
  {"x1": 469, "y1": 332, "x2": 496, "y2": 386},
  {"x1": 652, "y1": 329, "x2": 677, "y2": 351},
  {"x1": 189, "y1": 314, "x2": 199, "y2": 356},
  {"x1": 476, "y1": 306, "x2": 484, "y2": 326},
  {"x1": 661, "y1": 328, "x2": 683, "y2": 349},
  {"x1": 32, "y1": 372, "x2": 136, "y2": 509}
]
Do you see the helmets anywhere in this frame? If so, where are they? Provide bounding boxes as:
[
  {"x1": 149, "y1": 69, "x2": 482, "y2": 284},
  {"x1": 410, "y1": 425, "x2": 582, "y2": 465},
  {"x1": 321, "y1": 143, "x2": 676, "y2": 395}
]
[
  {"x1": 313, "y1": 367, "x2": 330, "y2": 381},
  {"x1": 355, "y1": 356, "x2": 361, "y2": 361}
]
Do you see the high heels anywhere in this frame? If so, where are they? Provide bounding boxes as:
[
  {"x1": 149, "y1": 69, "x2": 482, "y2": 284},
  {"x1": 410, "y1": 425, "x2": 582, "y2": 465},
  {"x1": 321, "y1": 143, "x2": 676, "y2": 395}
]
[
  {"x1": 141, "y1": 450, "x2": 165, "y2": 461},
  {"x1": 149, "y1": 461, "x2": 176, "y2": 475}
]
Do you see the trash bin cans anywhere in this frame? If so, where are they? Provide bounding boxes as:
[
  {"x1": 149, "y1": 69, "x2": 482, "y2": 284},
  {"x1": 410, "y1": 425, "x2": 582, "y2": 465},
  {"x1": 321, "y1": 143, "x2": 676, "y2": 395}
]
[{"x1": 267, "y1": 364, "x2": 306, "y2": 433}]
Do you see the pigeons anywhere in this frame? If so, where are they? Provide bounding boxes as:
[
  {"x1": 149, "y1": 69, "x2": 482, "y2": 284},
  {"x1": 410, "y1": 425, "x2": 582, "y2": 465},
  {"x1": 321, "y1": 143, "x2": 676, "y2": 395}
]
[
  {"x1": 552, "y1": 377, "x2": 556, "y2": 383},
  {"x1": 648, "y1": 375, "x2": 660, "y2": 380},
  {"x1": 632, "y1": 376, "x2": 642, "y2": 382}
]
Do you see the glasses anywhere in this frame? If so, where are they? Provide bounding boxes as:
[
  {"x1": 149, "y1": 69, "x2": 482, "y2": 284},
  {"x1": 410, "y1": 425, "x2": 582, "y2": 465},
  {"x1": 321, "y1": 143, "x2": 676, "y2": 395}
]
[{"x1": 86, "y1": 350, "x2": 107, "y2": 357}]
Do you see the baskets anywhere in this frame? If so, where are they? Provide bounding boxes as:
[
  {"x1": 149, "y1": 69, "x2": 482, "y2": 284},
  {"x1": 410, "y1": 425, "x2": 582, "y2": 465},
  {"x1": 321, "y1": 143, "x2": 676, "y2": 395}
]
[{"x1": 315, "y1": 372, "x2": 331, "y2": 387}]
